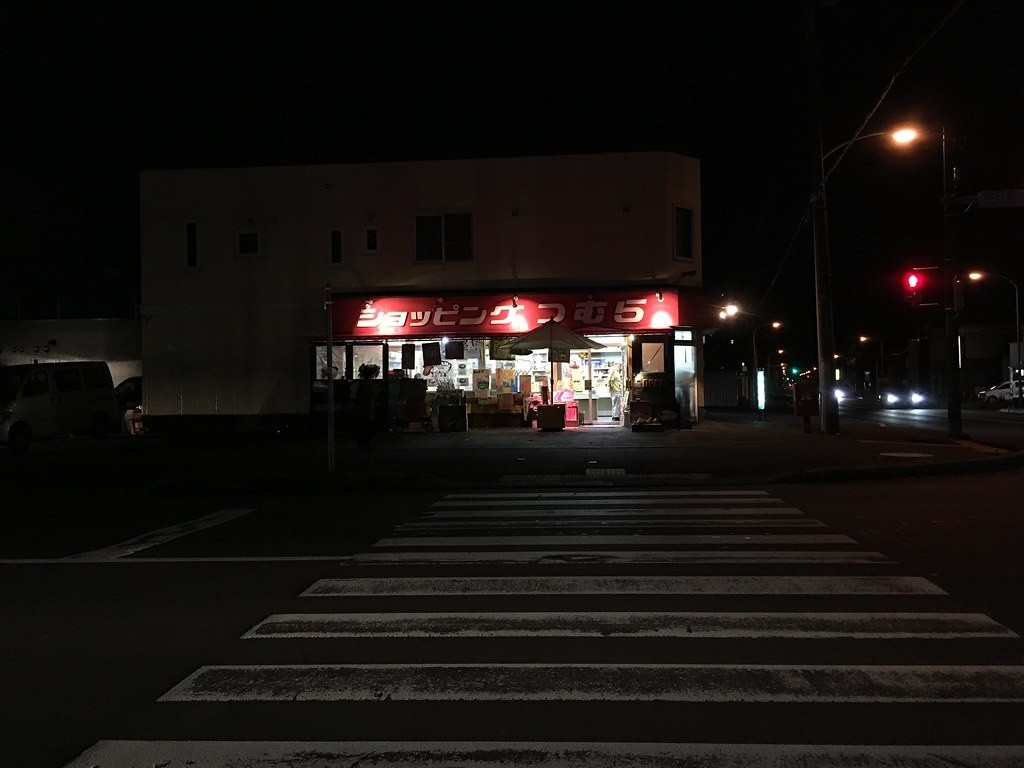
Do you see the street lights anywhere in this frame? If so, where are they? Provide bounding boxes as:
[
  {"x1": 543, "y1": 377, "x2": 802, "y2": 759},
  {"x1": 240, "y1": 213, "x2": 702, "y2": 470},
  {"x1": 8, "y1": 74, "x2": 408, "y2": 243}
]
[
  {"x1": 834, "y1": 354, "x2": 853, "y2": 394},
  {"x1": 859, "y1": 336, "x2": 884, "y2": 393},
  {"x1": 752, "y1": 321, "x2": 781, "y2": 422},
  {"x1": 814, "y1": 121, "x2": 924, "y2": 430},
  {"x1": 968, "y1": 272, "x2": 1023, "y2": 406},
  {"x1": 767, "y1": 349, "x2": 784, "y2": 415}
]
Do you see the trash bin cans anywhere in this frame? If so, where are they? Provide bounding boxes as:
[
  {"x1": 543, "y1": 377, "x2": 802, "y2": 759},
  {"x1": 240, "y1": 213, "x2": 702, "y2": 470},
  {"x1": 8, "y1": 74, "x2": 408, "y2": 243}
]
[
  {"x1": 793, "y1": 382, "x2": 819, "y2": 417},
  {"x1": 537, "y1": 404, "x2": 566, "y2": 432}
]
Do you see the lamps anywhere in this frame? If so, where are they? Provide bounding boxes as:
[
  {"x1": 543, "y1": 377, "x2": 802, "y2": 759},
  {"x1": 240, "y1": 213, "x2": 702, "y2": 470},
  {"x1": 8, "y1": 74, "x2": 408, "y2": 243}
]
[{"x1": 513, "y1": 299, "x2": 517, "y2": 307}]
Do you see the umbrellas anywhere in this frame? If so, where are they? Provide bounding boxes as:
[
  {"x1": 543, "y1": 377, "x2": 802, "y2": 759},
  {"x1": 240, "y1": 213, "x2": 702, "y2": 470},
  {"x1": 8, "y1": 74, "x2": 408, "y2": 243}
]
[{"x1": 498, "y1": 316, "x2": 608, "y2": 406}]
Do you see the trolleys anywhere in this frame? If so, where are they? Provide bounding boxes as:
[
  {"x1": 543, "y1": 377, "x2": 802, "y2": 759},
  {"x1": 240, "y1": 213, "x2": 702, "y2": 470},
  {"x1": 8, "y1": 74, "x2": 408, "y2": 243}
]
[{"x1": 391, "y1": 417, "x2": 433, "y2": 432}]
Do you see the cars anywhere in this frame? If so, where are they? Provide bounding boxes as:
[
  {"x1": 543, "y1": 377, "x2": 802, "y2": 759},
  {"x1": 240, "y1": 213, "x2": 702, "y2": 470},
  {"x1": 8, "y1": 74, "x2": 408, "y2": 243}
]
[
  {"x1": 879, "y1": 377, "x2": 927, "y2": 408},
  {"x1": 113, "y1": 376, "x2": 142, "y2": 413},
  {"x1": 977, "y1": 379, "x2": 1023, "y2": 404}
]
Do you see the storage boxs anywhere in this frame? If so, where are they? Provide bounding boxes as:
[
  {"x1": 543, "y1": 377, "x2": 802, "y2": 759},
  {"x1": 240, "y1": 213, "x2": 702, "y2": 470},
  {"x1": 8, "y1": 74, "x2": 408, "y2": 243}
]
[
  {"x1": 497, "y1": 393, "x2": 514, "y2": 411},
  {"x1": 631, "y1": 423, "x2": 663, "y2": 433},
  {"x1": 566, "y1": 406, "x2": 579, "y2": 427}
]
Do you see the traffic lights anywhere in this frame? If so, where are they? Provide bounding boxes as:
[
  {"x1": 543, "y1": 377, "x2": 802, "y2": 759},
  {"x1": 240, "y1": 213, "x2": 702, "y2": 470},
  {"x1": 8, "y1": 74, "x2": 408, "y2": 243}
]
[{"x1": 904, "y1": 271, "x2": 922, "y2": 302}]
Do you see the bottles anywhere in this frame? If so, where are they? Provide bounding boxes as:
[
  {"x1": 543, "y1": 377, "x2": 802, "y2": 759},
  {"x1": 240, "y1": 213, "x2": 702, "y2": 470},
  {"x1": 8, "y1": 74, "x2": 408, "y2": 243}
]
[{"x1": 131, "y1": 408, "x2": 143, "y2": 432}]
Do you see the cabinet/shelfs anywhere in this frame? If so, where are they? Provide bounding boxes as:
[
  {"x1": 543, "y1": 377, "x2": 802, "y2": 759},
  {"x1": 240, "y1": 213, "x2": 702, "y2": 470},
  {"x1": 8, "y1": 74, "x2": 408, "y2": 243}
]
[{"x1": 583, "y1": 349, "x2": 624, "y2": 398}]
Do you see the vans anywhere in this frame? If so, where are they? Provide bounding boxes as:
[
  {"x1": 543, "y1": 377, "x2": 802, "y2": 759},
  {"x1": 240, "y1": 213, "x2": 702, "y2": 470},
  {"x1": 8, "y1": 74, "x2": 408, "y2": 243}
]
[{"x1": 1, "y1": 360, "x2": 123, "y2": 451}]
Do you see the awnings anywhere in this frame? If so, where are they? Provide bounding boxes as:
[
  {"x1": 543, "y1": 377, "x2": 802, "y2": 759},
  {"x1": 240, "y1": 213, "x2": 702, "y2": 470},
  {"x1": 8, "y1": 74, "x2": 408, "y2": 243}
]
[{"x1": 329, "y1": 289, "x2": 681, "y2": 339}]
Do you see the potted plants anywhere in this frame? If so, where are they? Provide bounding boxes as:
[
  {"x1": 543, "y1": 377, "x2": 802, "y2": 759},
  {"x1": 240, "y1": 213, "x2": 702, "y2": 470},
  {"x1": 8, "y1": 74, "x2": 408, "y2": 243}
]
[{"x1": 358, "y1": 363, "x2": 380, "y2": 379}]
